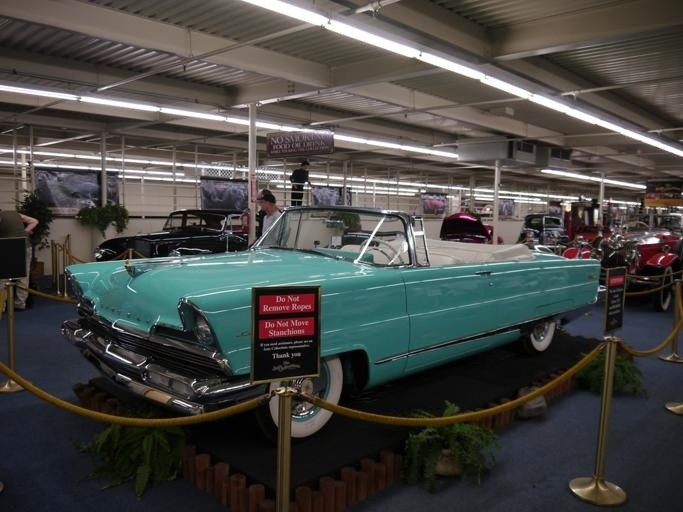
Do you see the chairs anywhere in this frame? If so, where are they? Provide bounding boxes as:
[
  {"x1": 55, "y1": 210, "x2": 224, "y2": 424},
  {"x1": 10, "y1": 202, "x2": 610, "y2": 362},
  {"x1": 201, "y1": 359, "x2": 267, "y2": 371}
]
[{"x1": 341, "y1": 235, "x2": 530, "y2": 266}]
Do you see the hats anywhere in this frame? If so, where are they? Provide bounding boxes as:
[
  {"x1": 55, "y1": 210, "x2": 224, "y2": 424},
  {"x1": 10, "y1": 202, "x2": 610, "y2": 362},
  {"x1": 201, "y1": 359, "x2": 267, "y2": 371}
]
[{"x1": 257, "y1": 189, "x2": 275, "y2": 203}]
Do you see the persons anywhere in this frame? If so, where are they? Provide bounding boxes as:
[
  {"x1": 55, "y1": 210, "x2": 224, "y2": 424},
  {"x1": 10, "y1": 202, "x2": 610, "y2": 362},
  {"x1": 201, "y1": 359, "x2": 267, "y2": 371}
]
[
  {"x1": 289, "y1": 159, "x2": 313, "y2": 206},
  {"x1": 258, "y1": 189, "x2": 282, "y2": 247},
  {"x1": 0, "y1": 208, "x2": 39, "y2": 312}
]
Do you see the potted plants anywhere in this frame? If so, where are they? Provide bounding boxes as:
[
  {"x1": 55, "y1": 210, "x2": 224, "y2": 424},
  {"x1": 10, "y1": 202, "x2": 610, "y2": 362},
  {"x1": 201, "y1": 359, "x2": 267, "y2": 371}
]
[
  {"x1": 76, "y1": 204, "x2": 129, "y2": 239},
  {"x1": 381, "y1": 399, "x2": 503, "y2": 495},
  {"x1": 330, "y1": 205, "x2": 359, "y2": 231},
  {"x1": 11, "y1": 186, "x2": 56, "y2": 279},
  {"x1": 572, "y1": 339, "x2": 649, "y2": 400}
]
